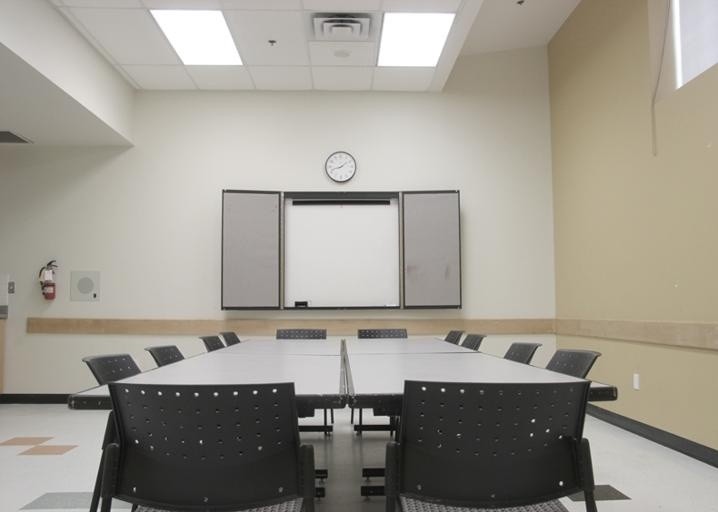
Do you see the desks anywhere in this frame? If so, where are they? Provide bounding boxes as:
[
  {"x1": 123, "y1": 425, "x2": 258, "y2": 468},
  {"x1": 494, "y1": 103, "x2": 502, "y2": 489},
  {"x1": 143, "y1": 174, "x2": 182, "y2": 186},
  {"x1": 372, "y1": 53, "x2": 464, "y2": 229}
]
[
  {"x1": 69, "y1": 355, "x2": 348, "y2": 499},
  {"x1": 345, "y1": 337, "x2": 476, "y2": 436},
  {"x1": 209, "y1": 337, "x2": 345, "y2": 435},
  {"x1": 344, "y1": 352, "x2": 617, "y2": 499}
]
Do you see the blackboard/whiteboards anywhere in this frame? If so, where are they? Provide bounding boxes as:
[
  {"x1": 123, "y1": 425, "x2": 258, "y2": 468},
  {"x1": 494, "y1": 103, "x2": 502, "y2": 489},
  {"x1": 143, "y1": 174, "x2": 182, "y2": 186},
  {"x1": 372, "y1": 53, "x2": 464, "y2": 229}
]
[{"x1": 222, "y1": 189, "x2": 461, "y2": 310}]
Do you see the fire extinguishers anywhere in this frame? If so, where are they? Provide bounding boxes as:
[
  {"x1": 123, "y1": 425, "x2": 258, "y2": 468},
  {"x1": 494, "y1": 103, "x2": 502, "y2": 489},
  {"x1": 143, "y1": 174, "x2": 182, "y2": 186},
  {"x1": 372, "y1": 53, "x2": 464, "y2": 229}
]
[{"x1": 39, "y1": 260, "x2": 58, "y2": 300}]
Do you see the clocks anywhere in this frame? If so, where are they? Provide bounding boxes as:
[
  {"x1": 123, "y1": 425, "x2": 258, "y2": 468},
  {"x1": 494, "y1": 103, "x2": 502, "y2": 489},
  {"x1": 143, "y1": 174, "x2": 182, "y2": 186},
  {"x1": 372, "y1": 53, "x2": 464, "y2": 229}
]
[{"x1": 324, "y1": 150, "x2": 356, "y2": 182}]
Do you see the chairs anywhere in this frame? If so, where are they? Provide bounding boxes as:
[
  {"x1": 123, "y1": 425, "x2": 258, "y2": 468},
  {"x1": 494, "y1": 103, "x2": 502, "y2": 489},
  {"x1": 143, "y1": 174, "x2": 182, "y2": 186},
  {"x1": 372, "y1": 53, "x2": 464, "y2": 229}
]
[
  {"x1": 146, "y1": 344, "x2": 184, "y2": 367},
  {"x1": 384, "y1": 379, "x2": 596, "y2": 511},
  {"x1": 81, "y1": 353, "x2": 141, "y2": 511},
  {"x1": 442, "y1": 330, "x2": 465, "y2": 346},
  {"x1": 219, "y1": 331, "x2": 240, "y2": 346},
  {"x1": 199, "y1": 335, "x2": 224, "y2": 353},
  {"x1": 100, "y1": 377, "x2": 317, "y2": 511},
  {"x1": 501, "y1": 342, "x2": 543, "y2": 365},
  {"x1": 461, "y1": 333, "x2": 487, "y2": 352},
  {"x1": 545, "y1": 349, "x2": 602, "y2": 379},
  {"x1": 358, "y1": 329, "x2": 408, "y2": 338},
  {"x1": 276, "y1": 329, "x2": 325, "y2": 340}
]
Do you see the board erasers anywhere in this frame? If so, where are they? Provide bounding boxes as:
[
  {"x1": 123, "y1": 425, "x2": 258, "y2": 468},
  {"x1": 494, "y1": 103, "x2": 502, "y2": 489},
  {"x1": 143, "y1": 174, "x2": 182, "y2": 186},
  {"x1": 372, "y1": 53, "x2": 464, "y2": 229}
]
[{"x1": 295, "y1": 301, "x2": 307, "y2": 308}]
